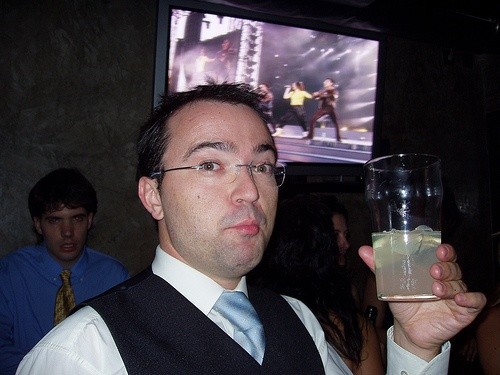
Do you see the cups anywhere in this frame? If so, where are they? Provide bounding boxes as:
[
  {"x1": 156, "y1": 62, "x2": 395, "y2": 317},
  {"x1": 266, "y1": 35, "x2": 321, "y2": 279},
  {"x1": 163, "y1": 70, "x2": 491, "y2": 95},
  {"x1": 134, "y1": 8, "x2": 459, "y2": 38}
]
[{"x1": 362, "y1": 151, "x2": 444, "y2": 302}]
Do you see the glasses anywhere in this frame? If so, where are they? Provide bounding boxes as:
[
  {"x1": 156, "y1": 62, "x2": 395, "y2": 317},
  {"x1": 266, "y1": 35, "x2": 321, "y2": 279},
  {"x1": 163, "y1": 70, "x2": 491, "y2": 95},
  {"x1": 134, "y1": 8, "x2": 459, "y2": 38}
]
[{"x1": 151, "y1": 157, "x2": 288, "y2": 190}]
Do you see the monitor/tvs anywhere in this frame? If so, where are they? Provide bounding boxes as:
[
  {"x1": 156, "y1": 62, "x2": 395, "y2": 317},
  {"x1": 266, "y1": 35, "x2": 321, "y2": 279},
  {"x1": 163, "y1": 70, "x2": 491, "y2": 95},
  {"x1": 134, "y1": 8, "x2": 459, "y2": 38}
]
[{"x1": 154, "y1": 0, "x2": 387, "y2": 176}]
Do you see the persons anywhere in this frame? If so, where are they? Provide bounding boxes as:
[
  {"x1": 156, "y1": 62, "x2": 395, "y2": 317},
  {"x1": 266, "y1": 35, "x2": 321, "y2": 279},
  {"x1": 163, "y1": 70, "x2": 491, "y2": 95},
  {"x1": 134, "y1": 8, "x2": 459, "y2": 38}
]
[
  {"x1": 191, "y1": 47, "x2": 215, "y2": 83},
  {"x1": 275, "y1": 198, "x2": 385, "y2": 374},
  {"x1": 461, "y1": 305, "x2": 500, "y2": 375},
  {"x1": 302, "y1": 79, "x2": 341, "y2": 143},
  {"x1": 258, "y1": 84, "x2": 276, "y2": 133},
  {"x1": 0, "y1": 169, "x2": 131, "y2": 375},
  {"x1": 272, "y1": 81, "x2": 319, "y2": 138},
  {"x1": 363, "y1": 269, "x2": 386, "y2": 366},
  {"x1": 216, "y1": 38, "x2": 237, "y2": 82},
  {"x1": 16, "y1": 83, "x2": 486, "y2": 375}
]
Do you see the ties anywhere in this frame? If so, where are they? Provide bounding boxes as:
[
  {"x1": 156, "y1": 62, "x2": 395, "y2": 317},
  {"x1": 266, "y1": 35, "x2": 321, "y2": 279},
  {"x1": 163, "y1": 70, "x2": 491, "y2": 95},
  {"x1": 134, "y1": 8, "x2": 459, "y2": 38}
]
[
  {"x1": 212, "y1": 291, "x2": 266, "y2": 365},
  {"x1": 54, "y1": 271, "x2": 76, "y2": 326}
]
